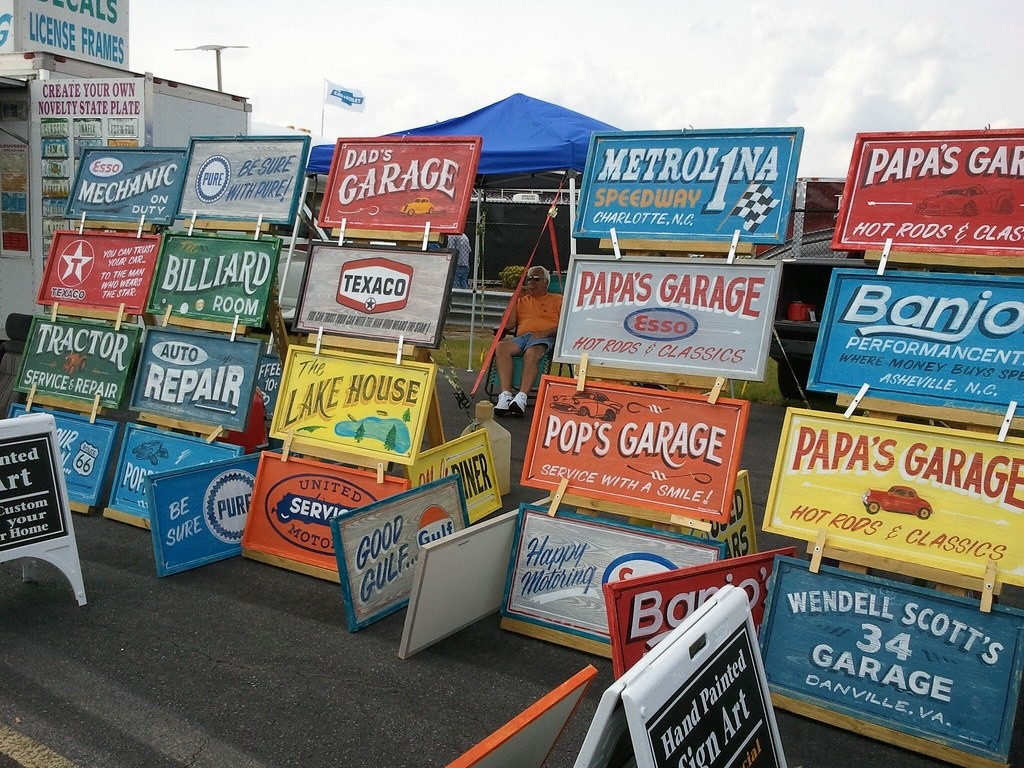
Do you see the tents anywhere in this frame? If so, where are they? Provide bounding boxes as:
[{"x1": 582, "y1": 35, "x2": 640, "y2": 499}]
[{"x1": 307, "y1": 92, "x2": 627, "y2": 372}]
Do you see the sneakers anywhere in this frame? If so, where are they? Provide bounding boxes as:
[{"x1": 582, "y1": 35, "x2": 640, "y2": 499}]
[
  {"x1": 493, "y1": 393, "x2": 514, "y2": 417},
  {"x1": 509, "y1": 394, "x2": 524, "y2": 418}
]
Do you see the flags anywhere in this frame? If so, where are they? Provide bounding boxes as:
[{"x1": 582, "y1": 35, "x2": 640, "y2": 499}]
[{"x1": 326, "y1": 80, "x2": 365, "y2": 112}]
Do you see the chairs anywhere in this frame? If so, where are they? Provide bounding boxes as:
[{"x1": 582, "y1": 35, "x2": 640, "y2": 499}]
[{"x1": 486, "y1": 273, "x2": 574, "y2": 406}]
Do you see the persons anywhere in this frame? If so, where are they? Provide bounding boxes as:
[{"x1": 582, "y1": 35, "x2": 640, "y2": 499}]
[
  {"x1": 446, "y1": 233, "x2": 472, "y2": 288},
  {"x1": 492, "y1": 266, "x2": 563, "y2": 417}
]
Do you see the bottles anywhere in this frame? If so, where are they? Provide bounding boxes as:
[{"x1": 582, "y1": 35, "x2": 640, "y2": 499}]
[{"x1": 460, "y1": 400, "x2": 512, "y2": 497}]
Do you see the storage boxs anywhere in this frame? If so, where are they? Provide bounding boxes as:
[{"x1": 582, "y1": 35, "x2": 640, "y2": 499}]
[{"x1": 787, "y1": 300, "x2": 817, "y2": 320}]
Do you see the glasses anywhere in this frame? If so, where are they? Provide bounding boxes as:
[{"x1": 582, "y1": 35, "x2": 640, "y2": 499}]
[{"x1": 526, "y1": 276, "x2": 544, "y2": 281}]
